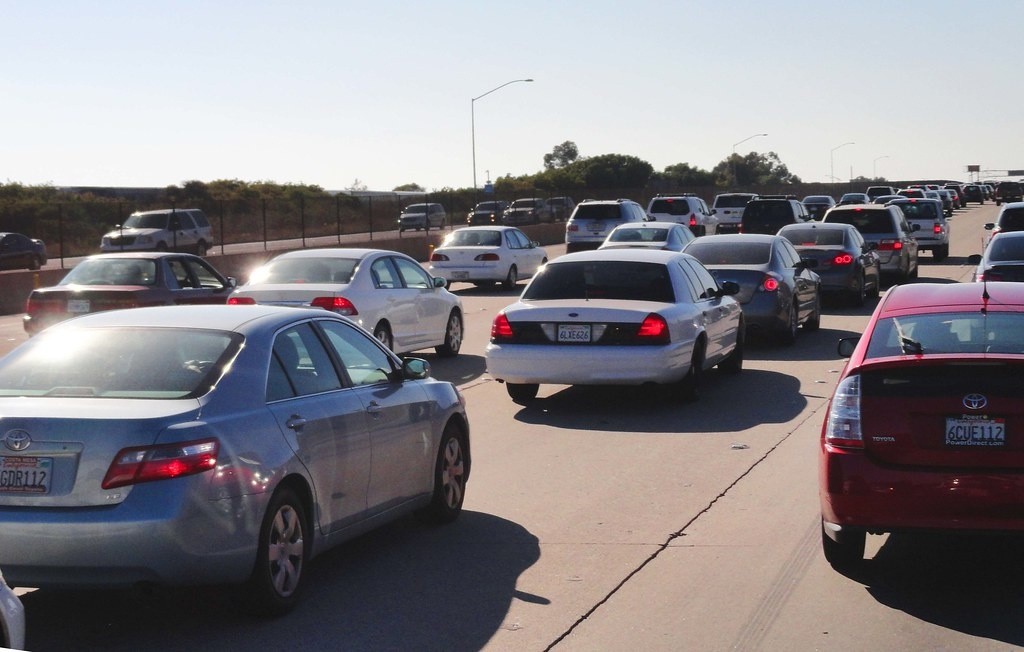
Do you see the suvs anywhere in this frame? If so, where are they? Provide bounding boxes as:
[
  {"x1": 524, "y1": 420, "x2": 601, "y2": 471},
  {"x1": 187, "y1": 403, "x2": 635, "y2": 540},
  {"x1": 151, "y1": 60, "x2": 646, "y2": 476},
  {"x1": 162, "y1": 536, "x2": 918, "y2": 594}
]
[
  {"x1": 866, "y1": 186, "x2": 894, "y2": 204},
  {"x1": 996, "y1": 182, "x2": 1023, "y2": 206},
  {"x1": 647, "y1": 194, "x2": 722, "y2": 239},
  {"x1": 823, "y1": 204, "x2": 922, "y2": 287},
  {"x1": 98, "y1": 207, "x2": 216, "y2": 260},
  {"x1": 711, "y1": 193, "x2": 762, "y2": 233},
  {"x1": 566, "y1": 198, "x2": 656, "y2": 256},
  {"x1": 503, "y1": 198, "x2": 554, "y2": 225},
  {"x1": 886, "y1": 198, "x2": 951, "y2": 263},
  {"x1": 467, "y1": 201, "x2": 506, "y2": 227},
  {"x1": 739, "y1": 194, "x2": 816, "y2": 240}
]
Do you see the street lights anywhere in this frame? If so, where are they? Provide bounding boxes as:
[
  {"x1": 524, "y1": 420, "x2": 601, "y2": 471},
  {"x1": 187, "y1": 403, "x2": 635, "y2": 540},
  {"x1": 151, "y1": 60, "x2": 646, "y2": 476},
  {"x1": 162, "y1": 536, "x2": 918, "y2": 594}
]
[
  {"x1": 831, "y1": 142, "x2": 855, "y2": 192},
  {"x1": 471, "y1": 79, "x2": 535, "y2": 225},
  {"x1": 873, "y1": 155, "x2": 890, "y2": 180},
  {"x1": 167, "y1": 187, "x2": 183, "y2": 253},
  {"x1": 732, "y1": 134, "x2": 768, "y2": 193}
]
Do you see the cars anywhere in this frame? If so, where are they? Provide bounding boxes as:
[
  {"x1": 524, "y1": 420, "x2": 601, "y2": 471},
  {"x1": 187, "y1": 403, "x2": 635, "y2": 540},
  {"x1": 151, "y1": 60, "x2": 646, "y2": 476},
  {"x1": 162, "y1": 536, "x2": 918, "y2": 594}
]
[
  {"x1": 598, "y1": 222, "x2": 696, "y2": 254},
  {"x1": 777, "y1": 222, "x2": 881, "y2": 305},
  {"x1": 873, "y1": 194, "x2": 907, "y2": 207},
  {"x1": 546, "y1": 196, "x2": 575, "y2": 222},
  {"x1": 224, "y1": 248, "x2": 465, "y2": 362},
  {"x1": 484, "y1": 248, "x2": 747, "y2": 402},
  {"x1": 429, "y1": 225, "x2": 548, "y2": 292},
  {"x1": 678, "y1": 235, "x2": 827, "y2": 350},
  {"x1": 973, "y1": 231, "x2": 1024, "y2": 284},
  {"x1": 819, "y1": 247, "x2": 1024, "y2": 573},
  {"x1": 801, "y1": 196, "x2": 835, "y2": 221},
  {"x1": 900, "y1": 179, "x2": 999, "y2": 217},
  {"x1": 984, "y1": 203, "x2": 1024, "y2": 249},
  {"x1": 0, "y1": 304, "x2": 475, "y2": 616},
  {"x1": 0, "y1": 231, "x2": 50, "y2": 272},
  {"x1": 397, "y1": 203, "x2": 447, "y2": 233},
  {"x1": 20, "y1": 252, "x2": 238, "y2": 340},
  {"x1": 840, "y1": 192, "x2": 873, "y2": 206},
  {"x1": 1017, "y1": 183, "x2": 1024, "y2": 196}
]
